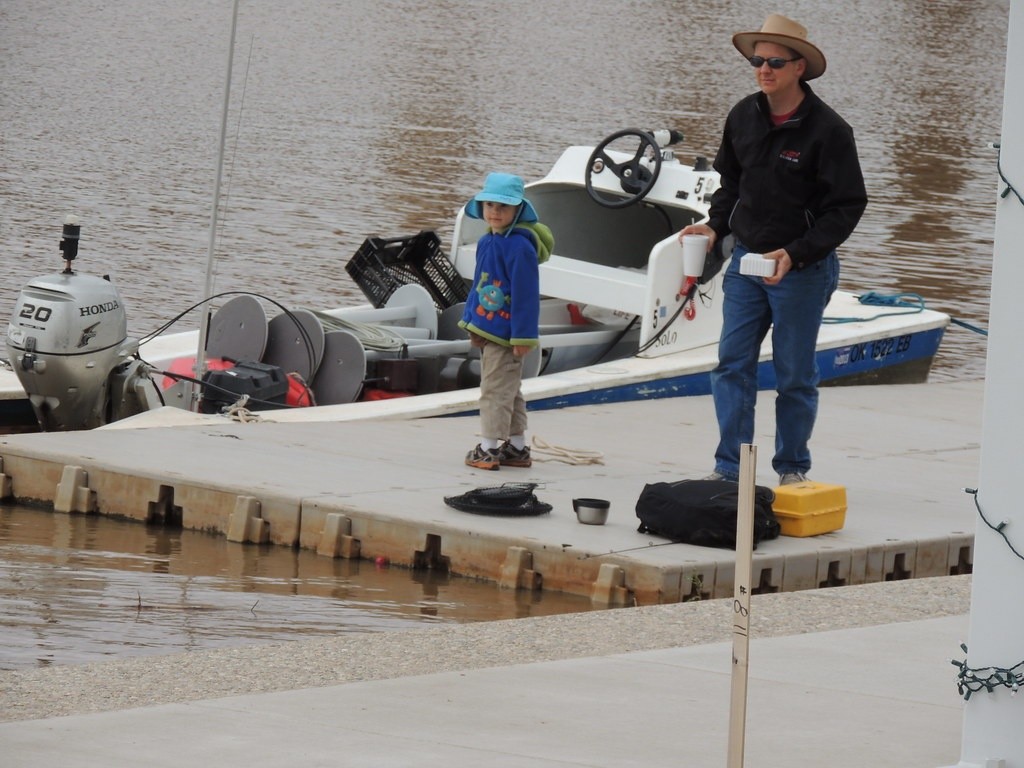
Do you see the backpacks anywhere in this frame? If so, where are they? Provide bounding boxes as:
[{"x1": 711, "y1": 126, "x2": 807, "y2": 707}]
[{"x1": 635, "y1": 479, "x2": 781, "y2": 551}]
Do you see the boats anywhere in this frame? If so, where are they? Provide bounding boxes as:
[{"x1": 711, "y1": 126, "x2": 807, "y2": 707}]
[{"x1": 1, "y1": 0, "x2": 954, "y2": 434}]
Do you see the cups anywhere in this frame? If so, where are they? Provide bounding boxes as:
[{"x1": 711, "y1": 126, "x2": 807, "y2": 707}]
[{"x1": 682, "y1": 233, "x2": 709, "y2": 277}]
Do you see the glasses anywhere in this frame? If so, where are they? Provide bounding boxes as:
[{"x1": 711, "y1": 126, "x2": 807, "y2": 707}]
[{"x1": 749, "y1": 55, "x2": 804, "y2": 69}]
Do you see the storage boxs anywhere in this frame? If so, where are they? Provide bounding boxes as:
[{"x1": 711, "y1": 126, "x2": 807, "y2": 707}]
[
  {"x1": 772, "y1": 480, "x2": 848, "y2": 538},
  {"x1": 204, "y1": 358, "x2": 291, "y2": 412}
]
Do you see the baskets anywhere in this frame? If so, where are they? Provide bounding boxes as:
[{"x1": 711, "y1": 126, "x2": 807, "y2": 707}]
[{"x1": 344, "y1": 230, "x2": 471, "y2": 311}]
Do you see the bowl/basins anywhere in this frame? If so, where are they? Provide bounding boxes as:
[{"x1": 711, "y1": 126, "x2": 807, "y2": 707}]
[{"x1": 573, "y1": 498, "x2": 611, "y2": 525}]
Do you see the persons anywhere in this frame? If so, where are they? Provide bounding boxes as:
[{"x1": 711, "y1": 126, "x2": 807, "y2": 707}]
[
  {"x1": 458, "y1": 173, "x2": 555, "y2": 470},
  {"x1": 678, "y1": 12, "x2": 868, "y2": 485}
]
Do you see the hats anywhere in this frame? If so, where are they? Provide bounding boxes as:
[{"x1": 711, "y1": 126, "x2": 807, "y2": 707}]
[
  {"x1": 732, "y1": 14, "x2": 826, "y2": 81},
  {"x1": 464, "y1": 172, "x2": 539, "y2": 224}
]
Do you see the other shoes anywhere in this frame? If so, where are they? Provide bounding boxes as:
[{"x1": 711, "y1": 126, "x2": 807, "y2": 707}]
[
  {"x1": 778, "y1": 472, "x2": 812, "y2": 486},
  {"x1": 701, "y1": 472, "x2": 737, "y2": 481}
]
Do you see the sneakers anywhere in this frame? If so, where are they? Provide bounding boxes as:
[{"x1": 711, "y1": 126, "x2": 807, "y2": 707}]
[
  {"x1": 497, "y1": 439, "x2": 532, "y2": 467},
  {"x1": 465, "y1": 443, "x2": 500, "y2": 470}
]
